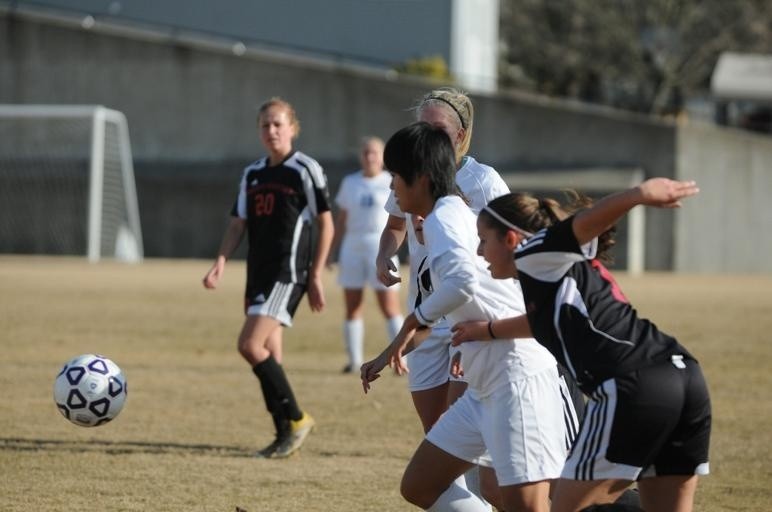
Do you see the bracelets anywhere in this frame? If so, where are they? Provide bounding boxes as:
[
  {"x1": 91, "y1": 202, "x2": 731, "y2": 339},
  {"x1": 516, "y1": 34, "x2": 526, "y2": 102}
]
[{"x1": 488, "y1": 320, "x2": 496, "y2": 339}]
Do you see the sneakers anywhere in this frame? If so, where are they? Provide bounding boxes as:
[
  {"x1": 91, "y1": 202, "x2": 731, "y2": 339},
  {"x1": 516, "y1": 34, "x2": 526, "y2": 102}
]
[
  {"x1": 256, "y1": 430, "x2": 301, "y2": 459},
  {"x1": 270, "y1": 411, "x2": 315, "y2": 458}
]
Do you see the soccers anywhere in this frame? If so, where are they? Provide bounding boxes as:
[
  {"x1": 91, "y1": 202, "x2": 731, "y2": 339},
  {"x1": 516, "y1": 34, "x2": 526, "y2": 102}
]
[{"x1": 53, "y1": 354, "x2": 128, "y2": 427}]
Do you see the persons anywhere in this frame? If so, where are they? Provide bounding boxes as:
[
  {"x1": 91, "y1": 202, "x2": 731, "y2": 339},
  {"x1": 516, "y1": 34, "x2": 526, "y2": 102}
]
[
  {"x1": 384, "y1": 121, "x2": 568, "y2": 511},
  {"x1": 202, "y1": 100, "x2": 336, "y2": 459},
  {"x1": 376, "y1": 90, "x2": 493, "y2": 511},
  {"x1": 324, "y1": 136, "x2": 408, "y2": 373},
  {"x1": 451, "y1": 176, "x2": 711, "y2": 512},
  {"x1": 360, "y1": 190, "x2": 586, "y2": 511}
]
